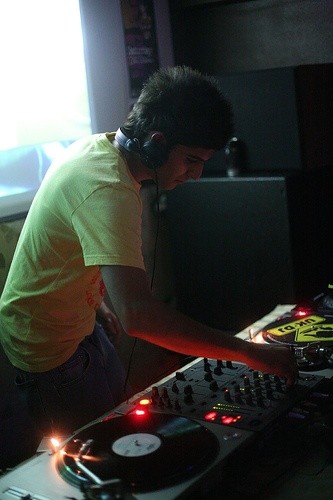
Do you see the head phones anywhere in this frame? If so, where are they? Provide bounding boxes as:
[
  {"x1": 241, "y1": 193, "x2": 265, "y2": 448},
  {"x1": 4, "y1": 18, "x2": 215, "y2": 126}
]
[{"x1": 113, "y1": 127, "x2": 170, "y2": 171}]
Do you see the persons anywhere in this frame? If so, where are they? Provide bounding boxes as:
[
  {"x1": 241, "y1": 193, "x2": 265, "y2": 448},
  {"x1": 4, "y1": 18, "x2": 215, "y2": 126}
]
[{"x1": 0, "y1": 68, "x2": 299, "y2": 452}]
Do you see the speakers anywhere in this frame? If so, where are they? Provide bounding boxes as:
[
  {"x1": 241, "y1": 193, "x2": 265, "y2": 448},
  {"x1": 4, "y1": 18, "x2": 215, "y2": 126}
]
[
  {"x1": 167, "y1": 175, "x2": 333, "y2": 331},
  {"x1": 197, "y1": 63, "x2": 333, "y2": 175}
]
